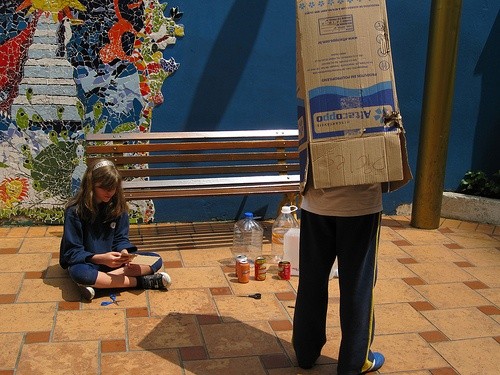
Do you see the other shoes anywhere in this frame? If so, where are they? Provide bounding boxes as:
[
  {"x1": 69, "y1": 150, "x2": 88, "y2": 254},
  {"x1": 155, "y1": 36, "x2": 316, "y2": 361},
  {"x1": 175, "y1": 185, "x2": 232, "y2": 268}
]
[{"x1": 367, "y1": 352, "x2": 385, "y2": 372}]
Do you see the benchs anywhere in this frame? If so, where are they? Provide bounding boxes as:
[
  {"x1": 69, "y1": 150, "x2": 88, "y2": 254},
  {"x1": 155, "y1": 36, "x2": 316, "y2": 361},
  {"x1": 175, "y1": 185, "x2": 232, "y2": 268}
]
[{"x1": 84, "y1": 129, "x2": 300, "y2": 221}]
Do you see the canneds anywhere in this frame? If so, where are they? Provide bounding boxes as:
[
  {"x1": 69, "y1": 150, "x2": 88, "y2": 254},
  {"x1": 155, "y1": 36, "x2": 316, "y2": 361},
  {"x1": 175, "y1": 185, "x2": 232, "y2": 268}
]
[
  {"x1": 254, "y1": 256, "x2": 267, "y2": 281},
  {"x1": 236, "y1": 255, "x2": 250, "y2": 283},
  {"x1": 278, "y1": 260, "x2": 292, "y2": 281}
]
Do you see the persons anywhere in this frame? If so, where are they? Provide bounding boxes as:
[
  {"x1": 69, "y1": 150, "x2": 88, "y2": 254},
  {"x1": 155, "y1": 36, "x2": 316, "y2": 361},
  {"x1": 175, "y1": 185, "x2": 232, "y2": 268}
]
[
  {"x1": 59, "y1": 159, "x2": 171, "y2": 302},
  {"x1": 290, "y1": 144, "x2": 385, "y2": 375}
]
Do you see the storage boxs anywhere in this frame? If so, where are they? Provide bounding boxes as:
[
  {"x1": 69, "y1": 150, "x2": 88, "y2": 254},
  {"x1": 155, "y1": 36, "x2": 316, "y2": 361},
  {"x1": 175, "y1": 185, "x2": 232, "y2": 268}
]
[{"x1": 295, "y1": 0, "x2": 414, "y2": 194}]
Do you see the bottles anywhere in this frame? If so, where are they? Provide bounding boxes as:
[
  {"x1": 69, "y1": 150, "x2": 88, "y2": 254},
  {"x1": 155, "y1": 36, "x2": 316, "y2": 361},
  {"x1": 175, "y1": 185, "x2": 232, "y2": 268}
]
[
  {"x1": 271, "y1": 205, "x2": 299, "y2": 263},
  {"x1": 233, "y1": 212, "x2": 264, "y2": 267}
]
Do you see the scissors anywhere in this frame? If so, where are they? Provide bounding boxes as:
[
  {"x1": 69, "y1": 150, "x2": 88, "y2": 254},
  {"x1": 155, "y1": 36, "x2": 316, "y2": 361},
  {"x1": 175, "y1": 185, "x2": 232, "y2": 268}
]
[
  {"x1": 237, "y1": 293, "x2": 261, "y2": 299},
  {"x1": 101, "y1": 295, "x2": 124, "y2": 305}
]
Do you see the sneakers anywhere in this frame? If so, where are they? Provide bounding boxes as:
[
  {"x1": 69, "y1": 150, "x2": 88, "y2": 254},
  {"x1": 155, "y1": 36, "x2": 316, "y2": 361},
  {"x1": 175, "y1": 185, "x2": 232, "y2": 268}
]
[
  {"x1": 81, "y1": 286, "x2": 95, "y2": 300},
  {"x1": 139, "y1": 272, "x2": 172, "y2": 289}
]
